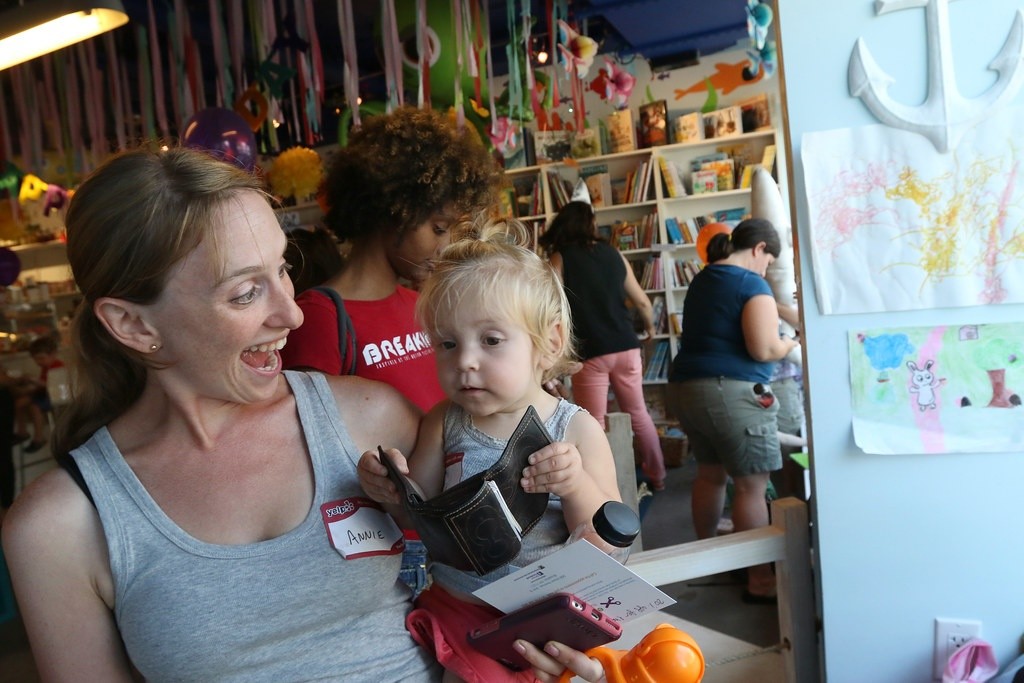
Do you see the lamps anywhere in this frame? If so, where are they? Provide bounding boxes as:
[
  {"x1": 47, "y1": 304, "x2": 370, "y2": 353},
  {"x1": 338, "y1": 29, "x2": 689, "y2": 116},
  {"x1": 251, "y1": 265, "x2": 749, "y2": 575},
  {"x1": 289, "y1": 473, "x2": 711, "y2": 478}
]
[{"x1": 0, "y1": 0, "x2": 129, "y2": 71}]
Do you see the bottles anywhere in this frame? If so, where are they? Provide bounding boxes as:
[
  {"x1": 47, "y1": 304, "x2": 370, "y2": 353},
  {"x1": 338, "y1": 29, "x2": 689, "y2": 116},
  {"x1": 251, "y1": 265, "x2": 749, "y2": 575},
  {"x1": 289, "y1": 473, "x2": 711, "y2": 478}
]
[
  {"x1": 562, "y1": 501, "x2": 641, "y2": 565},
  {"x1": 557, "y1": 624, "x2": 706, "y2": 683},
  {"x1": 753, "y1": 384, "x2": 774, "y2": 408}
]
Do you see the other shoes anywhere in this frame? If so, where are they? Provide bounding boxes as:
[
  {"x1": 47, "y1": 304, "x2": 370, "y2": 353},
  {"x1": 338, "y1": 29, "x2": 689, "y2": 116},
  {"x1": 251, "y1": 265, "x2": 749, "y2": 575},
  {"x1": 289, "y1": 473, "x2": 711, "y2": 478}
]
[
  {"x1": 739, "y1": 584, "x2": 781, "y2": 605},
  {"x1": 652, "y1": 478, "x2": 665, "y2": 493}
]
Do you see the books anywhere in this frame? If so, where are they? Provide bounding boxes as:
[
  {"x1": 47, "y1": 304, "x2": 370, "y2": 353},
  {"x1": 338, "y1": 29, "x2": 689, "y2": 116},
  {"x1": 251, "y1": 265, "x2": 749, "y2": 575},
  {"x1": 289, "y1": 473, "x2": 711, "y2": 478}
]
[
  {"x1": 658, "y1": 144, "x2": 776, "y2": 199},
  {"x1": 535, "y1": 95, "x2": 743, "y2": 162},
  {"x1": 529, "y1": 158, "x2": 654, "y2": 215},
  {"x1": 673, "y1": 255, "x2": 706, "y2": 287},
  {"x1": 614, "y1": 210, "x2": 670, "y2": 380},
  {"x1": 667, "y1": 206, "x2": 747, "y2": 246},
  {"x1": 672, "y1": 311, "x2": 683, "y2": 330}
]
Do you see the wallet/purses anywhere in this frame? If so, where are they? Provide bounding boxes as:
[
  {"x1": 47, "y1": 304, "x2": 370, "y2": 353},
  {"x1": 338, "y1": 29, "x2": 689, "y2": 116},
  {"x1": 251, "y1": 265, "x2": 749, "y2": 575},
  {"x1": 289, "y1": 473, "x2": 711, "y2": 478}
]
[{"x1": 377, "y1": 404, "x2": 555, "y2": 576}]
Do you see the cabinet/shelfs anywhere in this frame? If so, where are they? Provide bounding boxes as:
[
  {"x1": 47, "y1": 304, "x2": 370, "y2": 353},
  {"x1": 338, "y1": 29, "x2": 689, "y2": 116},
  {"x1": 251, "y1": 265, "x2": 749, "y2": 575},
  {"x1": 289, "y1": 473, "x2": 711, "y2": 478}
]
[{"x1": 480, "y1": 131, "x2": 779, "y2": 427}]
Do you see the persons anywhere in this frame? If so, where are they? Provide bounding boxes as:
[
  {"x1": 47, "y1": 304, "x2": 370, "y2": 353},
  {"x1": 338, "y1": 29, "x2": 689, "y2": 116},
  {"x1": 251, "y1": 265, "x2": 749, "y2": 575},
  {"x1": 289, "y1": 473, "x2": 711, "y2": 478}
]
[
  {"x1": 356, "y1": 218, "x2": 623, "y2": 683},
  {"x1": 0, "y1": 145, "x2": 607, "y2": 683},
  {"x1": 9, "y1": 337, "x2": 72, "y2": 453},
  {"x1": 535, "y1": 203, "x2": 669, "y2": 492},
  {"x1": 282, "y1": 107, "x2": 512, "y2": 423},
  {"x1": 665, "y1": 218, "x2": 801, "y2": 606},
  {"x1": 0, "y1": 371, "x2": 34, "y2": 653}
]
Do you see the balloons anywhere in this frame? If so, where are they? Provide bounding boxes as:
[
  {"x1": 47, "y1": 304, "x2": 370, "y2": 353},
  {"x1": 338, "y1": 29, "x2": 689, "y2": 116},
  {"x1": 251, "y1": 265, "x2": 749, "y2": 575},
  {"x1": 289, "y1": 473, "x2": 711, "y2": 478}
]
[
  {"x1": 180, "y1": 107, "x2": 257, "y2": 171},
  {"x1": 0, "y1": 247, "x2": 21, "y2": 287}
]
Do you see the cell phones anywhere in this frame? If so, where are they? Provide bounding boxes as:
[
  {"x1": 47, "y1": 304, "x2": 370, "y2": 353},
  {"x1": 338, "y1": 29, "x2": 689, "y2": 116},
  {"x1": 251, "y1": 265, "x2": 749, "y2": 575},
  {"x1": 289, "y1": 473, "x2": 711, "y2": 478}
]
[{"x1": 466, "y1": 592, "x2": 623, "y2": 673}]
